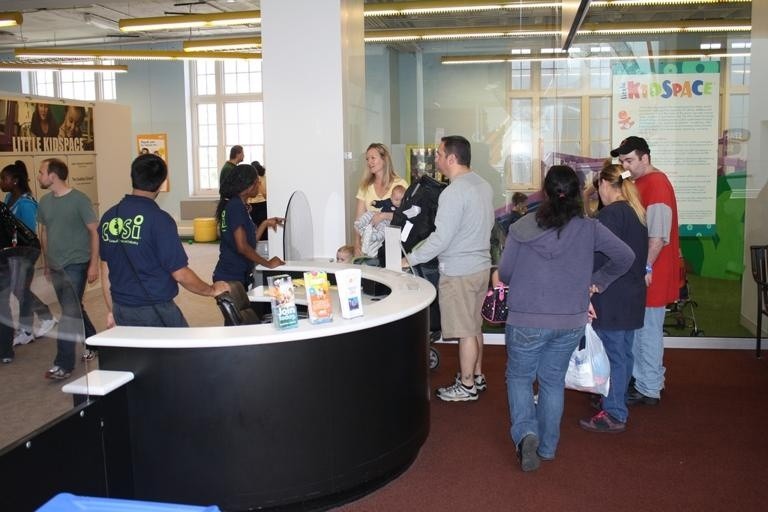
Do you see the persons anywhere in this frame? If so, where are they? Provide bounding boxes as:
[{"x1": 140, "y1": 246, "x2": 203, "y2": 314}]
[
  {"x1": 30, "y1": 102, "x2": 57, "y2": 137},
  {"x1": 57, "y1": 106, "x2": 86, "y2": 138}
]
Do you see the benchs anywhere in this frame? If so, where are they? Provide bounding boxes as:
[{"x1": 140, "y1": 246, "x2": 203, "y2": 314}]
[{"x1": 495, "y1": 201, "x2": 545, "y2": 254}]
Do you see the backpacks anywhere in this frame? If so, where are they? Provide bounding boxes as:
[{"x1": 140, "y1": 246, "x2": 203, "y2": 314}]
[{"x1": 0, "y1": 202, "x2": 41, "y2": 258}]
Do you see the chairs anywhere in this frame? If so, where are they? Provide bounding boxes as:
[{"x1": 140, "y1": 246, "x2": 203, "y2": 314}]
[
  {"x1": 750, "y1": 245, "x2": 768, "y2": 358},
  {"x1": 214, "y1": 280, "x2": 262, "y2": 326}
]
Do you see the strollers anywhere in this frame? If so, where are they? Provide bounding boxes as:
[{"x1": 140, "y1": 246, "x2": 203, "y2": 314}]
[
  {"x1": 349, "y1": 176, "x2": 452, "y2": 374},
  {"x1": 661, "y1": 274, "x2": 707, "y2": 337}
]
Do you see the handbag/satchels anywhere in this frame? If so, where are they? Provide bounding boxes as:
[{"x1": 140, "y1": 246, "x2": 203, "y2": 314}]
[
  {"x1": 563, "y1": 324, "x2": 612, "y2": 398},
  {"x1": 482, "y1": 281, "x2": 510, "y2": 327}
]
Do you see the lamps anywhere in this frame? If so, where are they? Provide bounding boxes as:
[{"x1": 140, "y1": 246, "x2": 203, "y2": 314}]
[
  {"x1": 363, "y1": 2, "x2": 752, "y2": 65},
  {"x1": 1, "y1": 11, "x2": 261, "y2": 74}
]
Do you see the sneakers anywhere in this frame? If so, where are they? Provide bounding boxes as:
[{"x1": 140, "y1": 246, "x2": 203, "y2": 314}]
[
  {"x1": 436, "y1": 380, "x2": 479, "y2": 403},
  {"x1": 45, "y1": 365, "x2": 73, "y2": 381},
  {"x1": 515, "y1": 433, "x2": 554, "y2": 472},
  {"x1": 11, "y1": 314, "x2": 67, "y2": 346},
  {"x1": 81, "y1": 347, "x2": 98, "y2": 363},
  {"x1": 622, "y1": 385, "x2": 664, "y2": 406},
  {"x1": 1, "y1": 351, "x2": 14, "y2": 365},
  {"x1": 576, "y1": 395, "x2": 628, "y2": 434},
  {"x1": 454, "y1": 371, "x2": 488, "y2": 392}
]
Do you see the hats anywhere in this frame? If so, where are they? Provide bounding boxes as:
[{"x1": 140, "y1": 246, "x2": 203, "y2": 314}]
[{"x1": 610, "y1": 135, "x2": 648, "y2": 156}]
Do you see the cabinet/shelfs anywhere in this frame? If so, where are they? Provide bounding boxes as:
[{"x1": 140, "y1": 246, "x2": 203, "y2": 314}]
[{"x1": 0, "y1": 152, "x2": 101, "y2": 269}]
